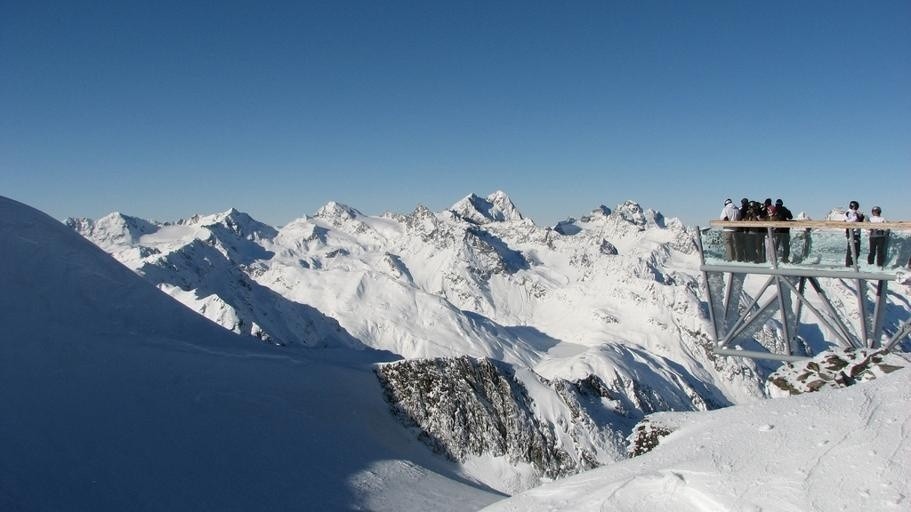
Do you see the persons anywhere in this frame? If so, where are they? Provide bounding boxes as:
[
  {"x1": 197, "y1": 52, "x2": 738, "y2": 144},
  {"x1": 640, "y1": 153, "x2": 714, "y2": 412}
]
[
  {"x1": 721, "y1": 196, "x2": 793, "y2": 263},
  {"x1": 843, "y1": 201, "x2": 867, "y2": 267},
  {"x1": 866, "y1": 207, "x2": 890, "y2": 270}
]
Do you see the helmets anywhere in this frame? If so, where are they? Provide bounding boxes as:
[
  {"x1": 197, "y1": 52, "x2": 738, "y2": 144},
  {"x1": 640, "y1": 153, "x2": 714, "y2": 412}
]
[
  {"x1": 872, "y1": 206, "x2": 882, "y2": 214},
  {"x1": 741, "y1": 198, "x2": 783, "y2": 209},
  {"x1": 724, "y1": 198, "x2": 731, "y2": 205},
  {"x1": 850, "y1": 201, "x2": 858, "y2": 209}
]
